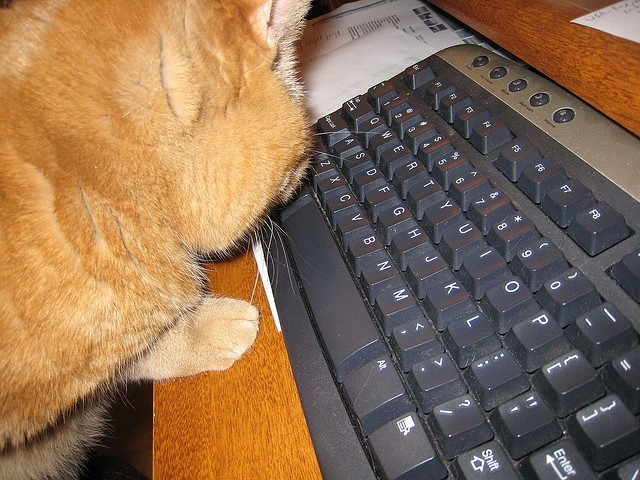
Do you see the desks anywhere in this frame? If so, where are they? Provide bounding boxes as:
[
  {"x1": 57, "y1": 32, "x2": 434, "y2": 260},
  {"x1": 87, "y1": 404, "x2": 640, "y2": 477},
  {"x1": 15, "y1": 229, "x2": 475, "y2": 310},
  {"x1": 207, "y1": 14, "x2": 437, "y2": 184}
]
[{"x1": 151, "y1": 3, "x2": 639, "y2": 480}]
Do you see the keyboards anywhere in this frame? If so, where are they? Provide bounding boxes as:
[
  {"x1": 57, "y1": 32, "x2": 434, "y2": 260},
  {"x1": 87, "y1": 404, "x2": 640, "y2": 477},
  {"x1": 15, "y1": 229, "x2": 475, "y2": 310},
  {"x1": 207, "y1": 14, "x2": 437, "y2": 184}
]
[{"x1": 259, "y1": 0, "x2": 640, "y2": 479}]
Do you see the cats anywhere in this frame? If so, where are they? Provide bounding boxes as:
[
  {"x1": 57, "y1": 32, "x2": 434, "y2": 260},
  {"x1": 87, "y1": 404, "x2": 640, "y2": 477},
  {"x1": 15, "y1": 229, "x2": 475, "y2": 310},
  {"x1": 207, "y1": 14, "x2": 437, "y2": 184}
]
[{"x1": 1, "y1": 0, "x2": 388, "y2": 480}]
